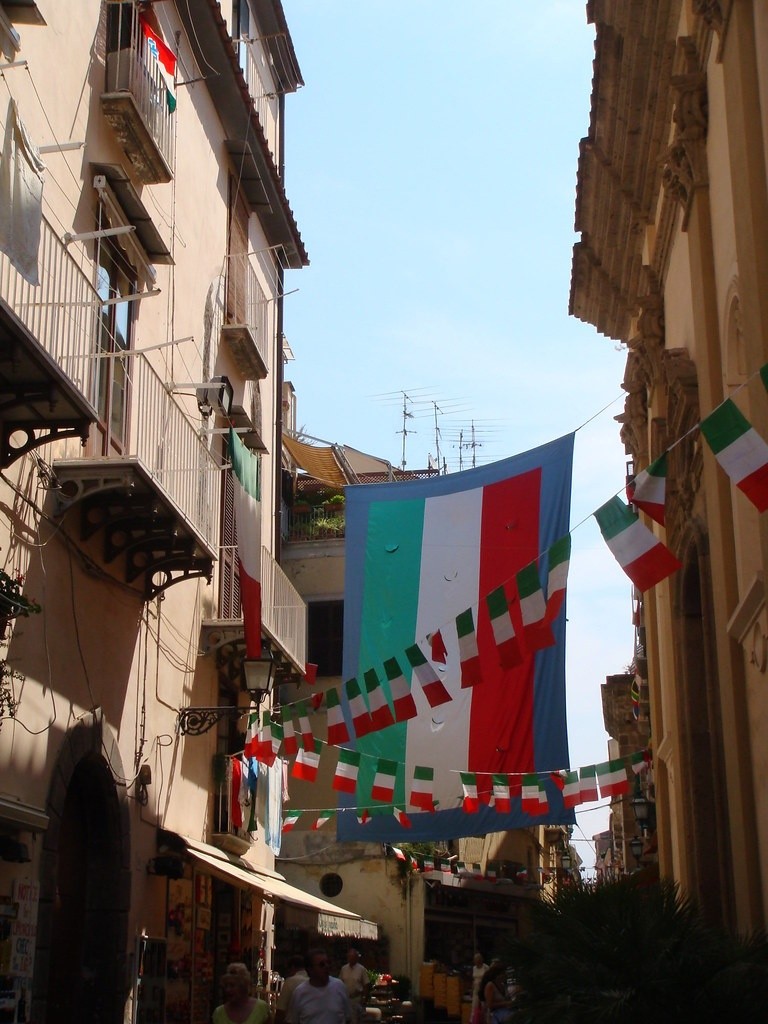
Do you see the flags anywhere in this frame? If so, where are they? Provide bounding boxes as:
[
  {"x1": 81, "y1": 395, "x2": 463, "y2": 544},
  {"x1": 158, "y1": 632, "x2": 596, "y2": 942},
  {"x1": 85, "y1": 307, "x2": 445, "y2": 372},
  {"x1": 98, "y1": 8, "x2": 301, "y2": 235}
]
[
  {"x1": 144, "y1": 23, "x2": 176, "y2": 114},
  {"x1": 625, "y1": 454, "x2": 668, "y2": 529},
  {"x1": 455, "y1": 608, "x2": 482, "y2": 689},
  {"x1": 700, "y1": 399, "x2": 768, "y2": 512},
  {"x1": 405, "y1": 643, "x2": 453, "y2": 708},
  {"x1": 425, "y1": 629, "x2": 448, "y2": 665},
  {"x1": 244, "y1": 669, "x2": 654, "y2": 887},
  {"x1": 231, "y1": 429, "x2": 262, "y2": 659},
  {"x1": 544, "y1": 536, "x2": 571, "y2": 624},
  {"x1": 486, "y1": 587, "x2": 524, "y2": 669},
  {"x1": 594, "y1": 496, "x2": 683, "y2": 593},
  {"x1": 516, "y1": 561, "x2": 556, "y2": 652},
  {"x1": 383, "y1": 657, "x2": 417, "y2": 723}
]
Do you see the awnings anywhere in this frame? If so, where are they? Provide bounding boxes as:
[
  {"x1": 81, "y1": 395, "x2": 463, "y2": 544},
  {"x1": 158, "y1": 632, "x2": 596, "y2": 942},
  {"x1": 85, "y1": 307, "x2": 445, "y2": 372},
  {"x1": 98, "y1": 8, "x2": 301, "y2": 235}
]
[{"x1": 185, "y1": 848, "x2": 380, "y2": 942}]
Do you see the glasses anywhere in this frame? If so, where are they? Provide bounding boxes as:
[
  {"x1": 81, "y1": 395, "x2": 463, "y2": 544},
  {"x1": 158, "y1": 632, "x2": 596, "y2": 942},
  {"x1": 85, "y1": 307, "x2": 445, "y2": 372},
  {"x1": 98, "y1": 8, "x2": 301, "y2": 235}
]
[{"x1": 312, "y1": 959, "x2": 333, "y2": 967}]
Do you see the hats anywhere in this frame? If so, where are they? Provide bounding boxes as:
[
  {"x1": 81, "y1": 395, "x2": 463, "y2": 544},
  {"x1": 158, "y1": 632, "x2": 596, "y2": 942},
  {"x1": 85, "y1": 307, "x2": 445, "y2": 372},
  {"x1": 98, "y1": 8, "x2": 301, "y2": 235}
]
[
  {"x1": 490, "y1": 959, "x2": 503, "y2": 969},
  {"x1": 227, "y1": 962, "x2": 250, "y2": 979}
]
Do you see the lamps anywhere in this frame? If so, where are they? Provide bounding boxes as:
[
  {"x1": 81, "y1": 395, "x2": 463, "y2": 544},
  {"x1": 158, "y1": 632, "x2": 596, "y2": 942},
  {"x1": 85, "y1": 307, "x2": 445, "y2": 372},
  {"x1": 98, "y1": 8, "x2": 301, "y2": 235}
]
[
  {"x1": 178, "y1": 640, "x2": 276, "y2": 737},
  {"x1": 195, "y1": 375, "x2": 234, "y2": 417}
]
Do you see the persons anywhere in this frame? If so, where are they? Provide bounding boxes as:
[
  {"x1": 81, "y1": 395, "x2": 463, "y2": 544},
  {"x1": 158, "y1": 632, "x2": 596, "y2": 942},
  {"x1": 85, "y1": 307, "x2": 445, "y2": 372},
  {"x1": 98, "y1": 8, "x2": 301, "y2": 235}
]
[
  {"x1": 340, "y1": 949, "x2": 370, "y2": 1024},
  {"x1": 275, "y1": 955, "x2": 310, "y2": 1024},
  {"x1": 291, "y1": 948, "x2": 352, "y2": 1024},
  {"x1": 212, "y1": 963, "x2": 272, "y2": 1024},
  {"x1": 470, "y1": 953, "x2": 521, "y2": 1024},
  {"x1": 504, "y1": 878, "x2": 768, "y2": 1024}
]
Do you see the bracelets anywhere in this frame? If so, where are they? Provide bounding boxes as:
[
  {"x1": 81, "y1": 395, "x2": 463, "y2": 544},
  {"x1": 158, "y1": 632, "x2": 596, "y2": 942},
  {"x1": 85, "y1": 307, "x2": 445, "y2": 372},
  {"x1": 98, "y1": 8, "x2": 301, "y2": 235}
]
[{"x1": 362, "y1": 996, "x2": 367, "y2": 999}]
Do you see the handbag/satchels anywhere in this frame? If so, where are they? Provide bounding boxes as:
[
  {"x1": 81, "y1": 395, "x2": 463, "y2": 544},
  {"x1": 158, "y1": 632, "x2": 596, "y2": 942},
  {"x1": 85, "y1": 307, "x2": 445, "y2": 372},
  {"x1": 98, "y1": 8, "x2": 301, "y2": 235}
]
[{"x1": 473, "y1": 1008, "x2": 485, "y2": 1024}]
